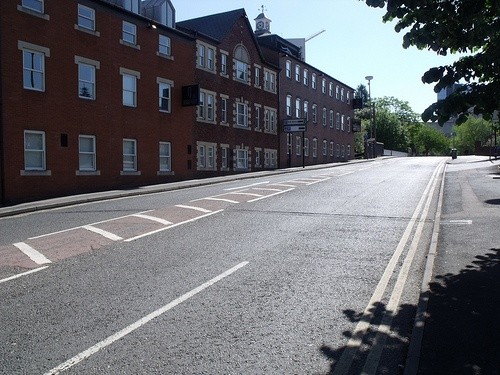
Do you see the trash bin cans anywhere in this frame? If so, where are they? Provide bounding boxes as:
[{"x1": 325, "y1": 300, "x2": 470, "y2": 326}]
[
  {"x1": 366, "y1": 137, "x2": 385, "y2": 158},
  {"x1": 452, "y1": 148, "x2": 457, "y2": 159}
]
